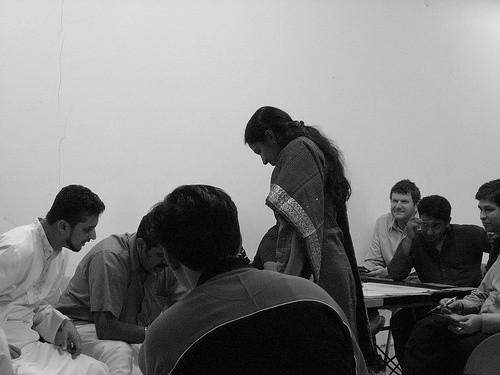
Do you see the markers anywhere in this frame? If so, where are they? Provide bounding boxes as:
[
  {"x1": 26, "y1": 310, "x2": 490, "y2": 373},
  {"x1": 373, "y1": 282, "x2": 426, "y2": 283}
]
[{"x1": 428, "y1": 296, "x2": 458, "y2": 314}]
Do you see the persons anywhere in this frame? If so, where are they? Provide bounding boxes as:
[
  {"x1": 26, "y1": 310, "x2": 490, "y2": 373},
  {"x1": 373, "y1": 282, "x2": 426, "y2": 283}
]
[
  {"x1": 55, "y1": 212, "x2": 187, "y2": 375},
  {"x1": 387, "y1": 196, "x2": 491, "y2": 368},
  {"x1": 0, "y1": 184, "x2": 109, "y2": 375},
  {"x1": 404, "y1": 180, "x2": 500, "y2": 375},
  {"x1": 252, "y1": 225, "x2": 278, "y2": 272},
  {"x1": 245, "y1": 106, "x2": 383, "y2": 375},
  {"x1": 357, "y1": 179, "x2": 421, "y2": 337},
  {"x1": 138, "y1": 184, "x2": 369, "y2": 375}
]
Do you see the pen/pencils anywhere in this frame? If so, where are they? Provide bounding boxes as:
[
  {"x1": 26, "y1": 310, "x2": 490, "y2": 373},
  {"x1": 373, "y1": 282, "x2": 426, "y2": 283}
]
[{"x1": 401, "y1": 211, "x2": 417, "y2": 237}]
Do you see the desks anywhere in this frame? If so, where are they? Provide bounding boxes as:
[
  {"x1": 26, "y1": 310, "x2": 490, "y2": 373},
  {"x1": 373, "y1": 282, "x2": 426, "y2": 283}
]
[{"x1": 361, "y1": 279, "x2": 478, "y2": 375}]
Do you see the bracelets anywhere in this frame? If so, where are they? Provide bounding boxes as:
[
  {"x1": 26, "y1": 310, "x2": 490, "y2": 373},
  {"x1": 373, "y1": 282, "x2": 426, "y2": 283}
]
[{"x1": 145, "y1": 326, "x2": 148, "y2": 336}]
